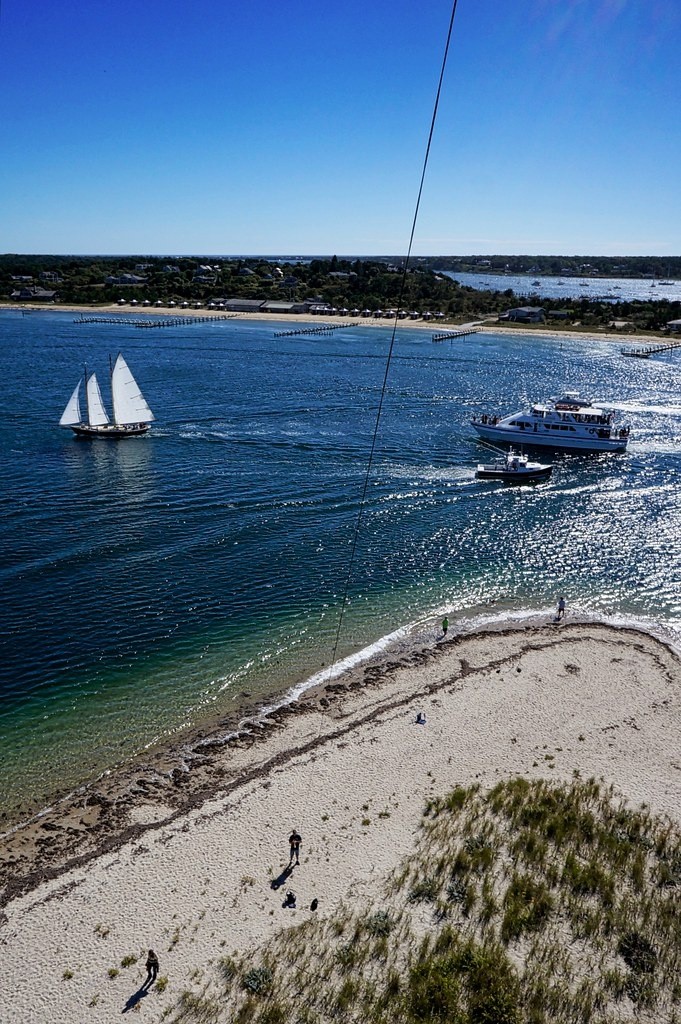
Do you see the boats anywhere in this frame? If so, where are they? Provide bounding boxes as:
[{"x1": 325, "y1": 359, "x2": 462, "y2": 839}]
[
  {"x1": 469, "y1": 436, "x2": 553, "y2": 483},
  {"x1": 469, "y1": 390, "x2": 631, "y2": 452}
]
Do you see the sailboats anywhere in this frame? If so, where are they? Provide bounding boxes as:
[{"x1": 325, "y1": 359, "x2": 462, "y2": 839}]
[{"x1": 57, "y1": 350, "x2": 160, "y2": 436}]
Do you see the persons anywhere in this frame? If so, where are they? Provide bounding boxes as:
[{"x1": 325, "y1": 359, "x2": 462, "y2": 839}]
[
  {"x1": 558, "y1": 597, "x2": 565, "y2": 617},
  {"x1": 416, "y1": 713, "x2": 421, "y2": 722},
  {"x1": 561, "y1": 414, "x2": 631, "y2": 439},
  {"x1": 288, "y1": 892, "x2": 296, "y2": 903},
  {"x1": 289, "y1": 829, "x2": 302, "y2": 865},
  {"x1": 145, "y1": 950, "x2": 159, "y2": 981},
  {"x1": 442, "y1": 616, "x2": 449, "y2": 636},
  {"x1": 473, "y1": 413, "x2": 503, "y2": 425}
]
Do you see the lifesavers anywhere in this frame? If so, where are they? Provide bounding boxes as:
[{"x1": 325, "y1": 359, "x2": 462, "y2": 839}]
[
  {"x1": 514, "y1": 467, "x2": 518, "y2": 472},
  {"x1": 588, "y1": 428, "x2": 595, "y2": 435}
]
